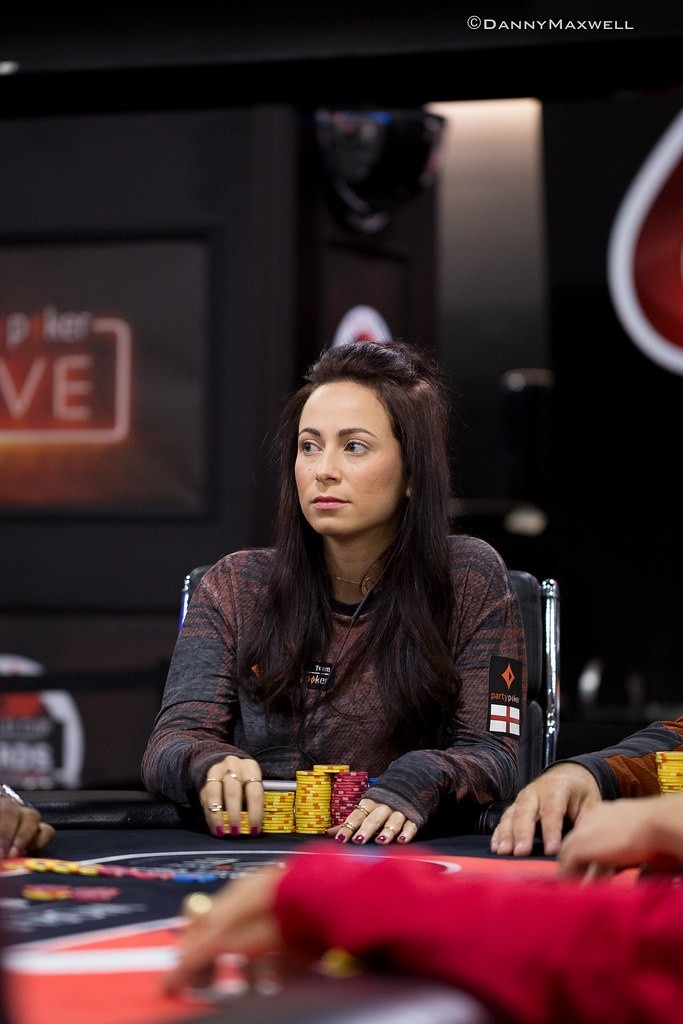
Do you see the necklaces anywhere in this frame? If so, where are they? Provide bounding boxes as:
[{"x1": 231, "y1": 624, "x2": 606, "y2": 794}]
[{"x1": 330, "y1": 573, "x2": 380, "y2": 592}]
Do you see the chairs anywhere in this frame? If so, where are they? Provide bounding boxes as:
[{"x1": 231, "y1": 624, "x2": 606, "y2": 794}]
[{"x1": 181, "y1": 567, "x2": 562, "y2": 769}]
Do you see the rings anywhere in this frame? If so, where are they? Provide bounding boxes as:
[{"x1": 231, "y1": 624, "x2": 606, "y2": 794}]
[
  {"x1": 220, "y1": 769, "x2": 242, "y2": 783},
  {"x1": 340, "y1": 821, "x2": 357, "y2": 834},
  {"x1": 242, "y1": 776, "x2": 263, "y2": 787},
  {"x1": 384, "y1": 824, "x2": 396, "y2": 836},
  {"x1": 352, "y1": 803, "x2": 370, "y2": 817},
  {"x1": 207, "y1": 802, "x2": 222, "y2": 813},
  {"x1": 205, "y1": 777, "x2": 223, "y2": 784}
]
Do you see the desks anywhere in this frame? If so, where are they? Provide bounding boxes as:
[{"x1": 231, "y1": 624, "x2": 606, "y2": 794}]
[{"x1": 37, "y1": 828, "x2": 554, "y2": 867}]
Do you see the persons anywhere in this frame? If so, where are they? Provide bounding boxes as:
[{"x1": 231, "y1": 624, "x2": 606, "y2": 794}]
[
  {"x1": 138, "y1": 340, "x2": 529, "y2": 849},
  {"x1": 484, "y1": 717, "x2": 682, "y2": 880},
  {"x1": 0, "y1": 785, "x2": 55, "y2": 859},
  {"x1": 161, "y1": 842, "x2": 682, "y2": 1023}
]
